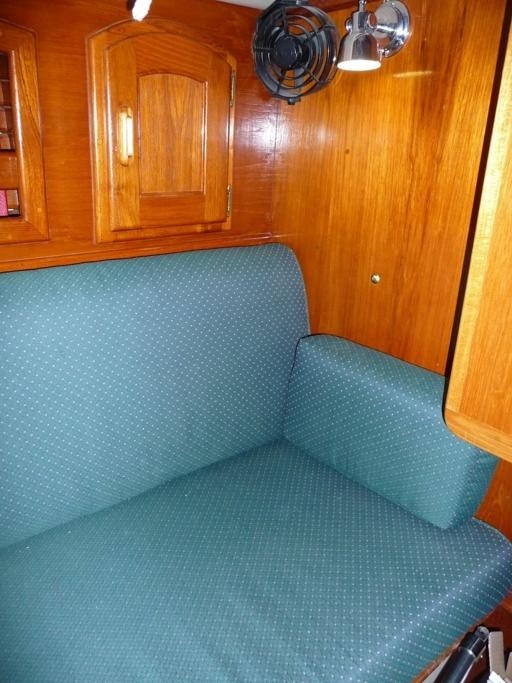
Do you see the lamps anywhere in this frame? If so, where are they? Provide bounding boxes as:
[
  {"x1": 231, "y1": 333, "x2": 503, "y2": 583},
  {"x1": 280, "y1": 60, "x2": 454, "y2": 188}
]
[
  {"x1": 125, "y1": 0, "x2": 158, "y2": 24},
  {"x1": 335, "y1": 0, "x2": 410, "y2": 72}
]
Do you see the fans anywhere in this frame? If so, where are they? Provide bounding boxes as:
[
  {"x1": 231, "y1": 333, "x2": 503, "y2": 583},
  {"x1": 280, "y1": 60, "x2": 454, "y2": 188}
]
[{"x1": 251, "y1": 0, "x2": 342, "y2": 107}]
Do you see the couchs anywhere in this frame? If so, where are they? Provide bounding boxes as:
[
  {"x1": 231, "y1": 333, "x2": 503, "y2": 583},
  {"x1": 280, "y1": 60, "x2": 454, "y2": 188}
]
[{"x1": 0, "y1": 240, "x2": 511, "y2": 682}]
